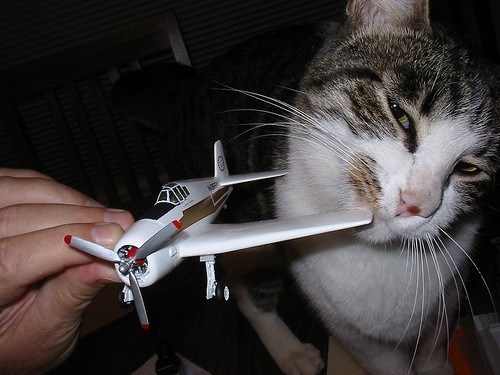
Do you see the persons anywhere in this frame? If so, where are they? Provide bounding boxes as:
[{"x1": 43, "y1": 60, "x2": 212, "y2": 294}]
[{"x1": 1, "y1": 166, "x2": 136, "y2": 374}]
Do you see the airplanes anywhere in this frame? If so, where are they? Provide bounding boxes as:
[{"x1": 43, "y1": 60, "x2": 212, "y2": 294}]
[{"x1": 65, "y1": 140, "x2": 373, "y2": 330}]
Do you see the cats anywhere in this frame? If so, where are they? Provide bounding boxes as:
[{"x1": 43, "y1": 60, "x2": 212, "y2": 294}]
[{"x1": 108, "y1": 1, "x2": 500, "y2": 375}]
